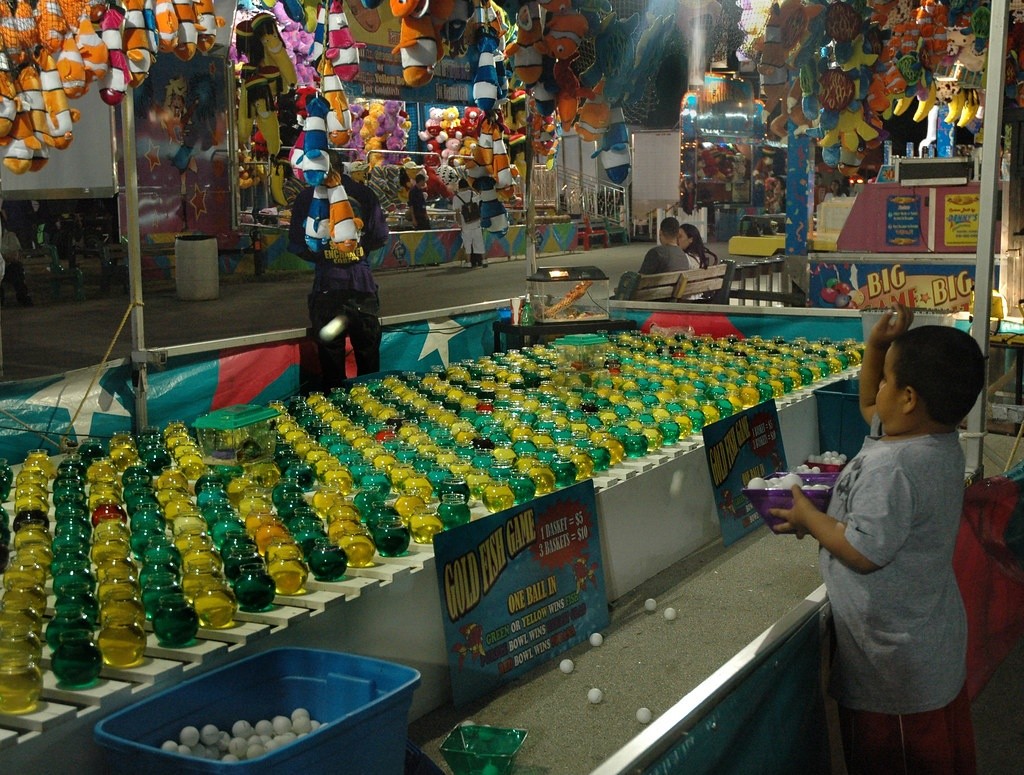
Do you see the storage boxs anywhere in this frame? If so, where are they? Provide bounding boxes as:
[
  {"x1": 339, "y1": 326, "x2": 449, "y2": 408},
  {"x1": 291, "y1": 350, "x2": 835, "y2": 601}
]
[
  {"x1": 527, "y1": 266, "x2": 610, "y2": 323},
  {"x1": 814, "y1": 379, "x2": 871, "y2": 461},
  {"x1": 94, "y1": 646, "x2": 423, "y2": 775},
  {"x1": 193, "y1": 404, "x2": 280, "y2": 469}
]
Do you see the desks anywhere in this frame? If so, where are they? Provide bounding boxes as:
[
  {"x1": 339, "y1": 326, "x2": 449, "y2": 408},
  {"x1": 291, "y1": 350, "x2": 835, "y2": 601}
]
[{"x1": 494, "y1": 318, "x2": 637, "y2": 351}]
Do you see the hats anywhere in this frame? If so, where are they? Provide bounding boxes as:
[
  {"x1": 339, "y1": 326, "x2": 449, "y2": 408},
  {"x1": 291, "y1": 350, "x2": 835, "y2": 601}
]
[{"x1": 459, "y1": 179, "x2": 469, "y2": 188}]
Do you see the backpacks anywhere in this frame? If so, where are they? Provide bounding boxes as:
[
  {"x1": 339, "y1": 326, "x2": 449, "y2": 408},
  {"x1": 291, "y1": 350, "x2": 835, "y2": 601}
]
[{"x1": 455, "y1": 190, "x2": 480, "y2": 223}]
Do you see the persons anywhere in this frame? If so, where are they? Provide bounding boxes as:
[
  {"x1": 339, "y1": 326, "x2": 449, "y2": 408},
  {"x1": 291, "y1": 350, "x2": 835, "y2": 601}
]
[
  {"x1": 408, "y1": 174, "x2": 430, "y2": 230},
  {"x1": 453, "y1": 179, "x2": 488, "y2": 268},
  {"x1": 40, "y1": 211, "x2": 83, "y2": 271},
  {"x1": 0, "y1": 222, "x2": 34, "y2": 310},
  {"x1": 288, "y1": 150, "x2": 389, "y2": 398},
  {"x1": 813, "y1": 171, "x2": 846, "y2": 213},
  {"x1": 770, "y1": 301, "x2": 988, "y2": 775},
  {"x1": 639, "y1": 217, "x2": 706, "y2": 274}
]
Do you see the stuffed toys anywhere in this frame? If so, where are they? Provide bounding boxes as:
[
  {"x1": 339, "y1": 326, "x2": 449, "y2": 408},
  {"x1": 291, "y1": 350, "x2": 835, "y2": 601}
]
[{"x1": 0, "y1": 0, "x2": 866, "y2": 228}]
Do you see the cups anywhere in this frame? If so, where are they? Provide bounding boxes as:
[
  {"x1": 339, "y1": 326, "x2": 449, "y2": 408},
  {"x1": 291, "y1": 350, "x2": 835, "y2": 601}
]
[{"x1": 509, "y1": 298, "x2": 522, "y2": 325}]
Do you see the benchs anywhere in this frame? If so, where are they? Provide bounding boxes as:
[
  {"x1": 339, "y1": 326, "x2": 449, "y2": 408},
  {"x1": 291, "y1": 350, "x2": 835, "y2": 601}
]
[
  {"x1": 613, "y1": 259, "x2": 736, "y2": 304},
  {"x1": 18, "y1": 244, "x2": 85, "y2": 303},
  {"x1": 98, "y1": 240, "x2": 175, "y2": 298}
]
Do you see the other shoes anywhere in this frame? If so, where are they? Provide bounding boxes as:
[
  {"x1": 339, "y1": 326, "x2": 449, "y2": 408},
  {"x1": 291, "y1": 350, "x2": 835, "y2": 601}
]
[
  {"x1": 18, "y1": 299, "x2": 33, "y2": 307},
  {"x1": 478, "y1": 263, "x2": 482, "y2": 266},
  {"x1": 472, "y1": 263, "x2": 476, "y2": 267}
]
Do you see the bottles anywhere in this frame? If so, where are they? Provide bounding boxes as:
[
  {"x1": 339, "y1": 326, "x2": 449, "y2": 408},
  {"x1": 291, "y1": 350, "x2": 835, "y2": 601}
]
[
  {"x1": 884, "y1": 140, "x2": 974, "y2": 165},
  {"x1": 522, "y1": 293, "x2": 533, "y2": 326},
  {"x1": 0, "y1": 330, "x2": 870, "y2": 716}
]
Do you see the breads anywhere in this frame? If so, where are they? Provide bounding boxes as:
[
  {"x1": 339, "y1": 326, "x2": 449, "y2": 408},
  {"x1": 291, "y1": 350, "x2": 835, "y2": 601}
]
[{"x1": 542, "y1": 280, "x2": 593, "y2": 318}]
